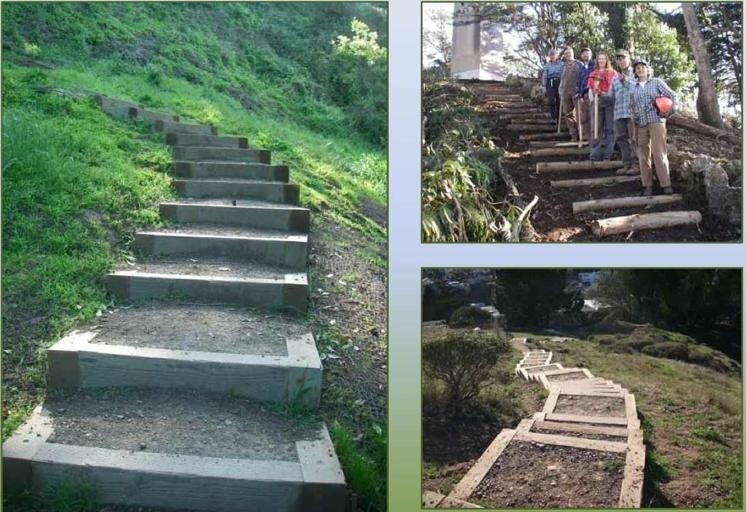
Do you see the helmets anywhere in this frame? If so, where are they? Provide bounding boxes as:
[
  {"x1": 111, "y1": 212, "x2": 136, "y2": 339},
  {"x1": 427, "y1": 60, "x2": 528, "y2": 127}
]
[{"x1": 656, "y1": 97, "x2": 672, "y2": 112}]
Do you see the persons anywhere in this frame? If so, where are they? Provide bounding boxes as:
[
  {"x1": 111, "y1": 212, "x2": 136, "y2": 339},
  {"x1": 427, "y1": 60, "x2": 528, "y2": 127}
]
[
  {"x1": 588, "y1": 50, "x2": 618, "y2": 161},
  {"x1": 593, "y1": 49, "x2": 640, "y2": 176},
  {"x1": 558, "y1": 46, "x2": 585, "y2": 142},
  {"x1": 542, "y1": 49, "x2": 565, "y2": 124},
  {"x1": 575, "y1": 47, "x2": 599, "y2": 140},
  {"x1": 629, "y1": 58, "x2": 676, "y2": 195}
]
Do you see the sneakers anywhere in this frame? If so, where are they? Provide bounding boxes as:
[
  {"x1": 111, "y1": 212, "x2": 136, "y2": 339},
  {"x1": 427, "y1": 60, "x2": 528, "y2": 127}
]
[{"x1": 590, "y1": 153, "x2": 673, "y2": 195}]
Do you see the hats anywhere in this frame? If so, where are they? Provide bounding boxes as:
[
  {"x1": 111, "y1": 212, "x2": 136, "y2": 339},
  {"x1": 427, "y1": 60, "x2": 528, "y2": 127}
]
[{"x1": 564, "y1": 46, "x2": 647, "y2": 65}]
